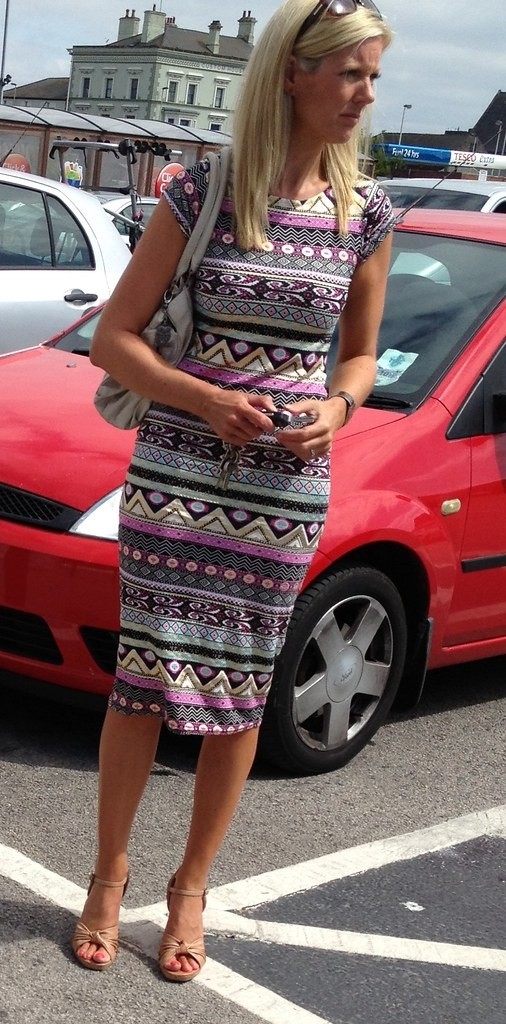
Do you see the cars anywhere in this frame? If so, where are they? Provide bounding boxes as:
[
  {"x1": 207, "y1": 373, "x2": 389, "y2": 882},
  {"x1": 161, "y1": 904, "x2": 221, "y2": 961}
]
[
  {"x1": 377, "y1": 173, "x2": 506, "y2": 215},
  {"x1": 0, "y1": 206, "x2": 506, "y2": 773},
  {"x1": 106, "y1": 195, "x2": 162, "y2": 252},
  {"x1": 1, "y1": 163, "x2": 134, "y2": 359}
]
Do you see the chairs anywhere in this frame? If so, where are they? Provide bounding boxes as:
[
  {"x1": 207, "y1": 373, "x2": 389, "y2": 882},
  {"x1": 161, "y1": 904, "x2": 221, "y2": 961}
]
[
  {"x1": 381, "y1": 274, "x2": 434, "y2": 330},
  {"x1": 393, "y1": 282, "x2": 477, "y2": 378},
  {"x1": 30, "y1": 216, "x2": 62, "y2": 266}
]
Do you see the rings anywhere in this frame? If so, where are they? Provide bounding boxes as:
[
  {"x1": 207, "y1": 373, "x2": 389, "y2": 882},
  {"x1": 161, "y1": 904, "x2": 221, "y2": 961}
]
[{"x1": 311, "y1": 450, "x2": 315, "y2": 459}]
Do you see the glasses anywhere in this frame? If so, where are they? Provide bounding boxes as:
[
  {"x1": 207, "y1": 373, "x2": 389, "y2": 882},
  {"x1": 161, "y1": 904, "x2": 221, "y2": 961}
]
[{"x1": 294, "y1": 0, "x2": 382, "y2": 45}]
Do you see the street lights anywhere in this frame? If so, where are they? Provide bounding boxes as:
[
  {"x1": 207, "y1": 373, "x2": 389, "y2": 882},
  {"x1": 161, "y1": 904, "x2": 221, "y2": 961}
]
[
  {"x1": 398, "y1": 105, "x2": 413, "y2": 146},
  {"x1": 493, "y1": 119, "x2": 503, "y2": 154}
]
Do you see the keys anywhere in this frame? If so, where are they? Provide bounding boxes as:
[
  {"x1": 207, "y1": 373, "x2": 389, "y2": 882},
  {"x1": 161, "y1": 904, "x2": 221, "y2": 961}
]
[{"x1": 215, "y1": 411, "x2": 315, "y2": 489}]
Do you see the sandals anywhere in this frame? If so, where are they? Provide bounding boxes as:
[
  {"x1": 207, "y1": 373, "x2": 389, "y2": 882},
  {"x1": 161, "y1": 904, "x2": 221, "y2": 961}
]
[
  {"x1": 71, "y1": 869, "x2": 133, "y2": 969},
  {"x1": 156, "y1": 869, "x2": 208, "y2": 984}
]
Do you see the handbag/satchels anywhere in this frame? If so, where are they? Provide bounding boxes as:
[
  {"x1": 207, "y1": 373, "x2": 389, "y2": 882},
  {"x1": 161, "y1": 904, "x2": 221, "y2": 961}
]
[{"x1": 94, "y1": 145, "x2": 233, "y2": 431}]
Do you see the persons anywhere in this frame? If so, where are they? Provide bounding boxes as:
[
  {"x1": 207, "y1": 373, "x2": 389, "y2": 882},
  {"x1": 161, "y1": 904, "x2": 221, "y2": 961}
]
[{"x1": 74, "y1": 0, "x2": 392, "y2": 982}]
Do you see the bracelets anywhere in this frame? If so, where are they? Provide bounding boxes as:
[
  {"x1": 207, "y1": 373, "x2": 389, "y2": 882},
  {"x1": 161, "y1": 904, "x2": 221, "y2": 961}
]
[{"x1": 327, "y1": 391, "x2": 355, "y2": 428}]
